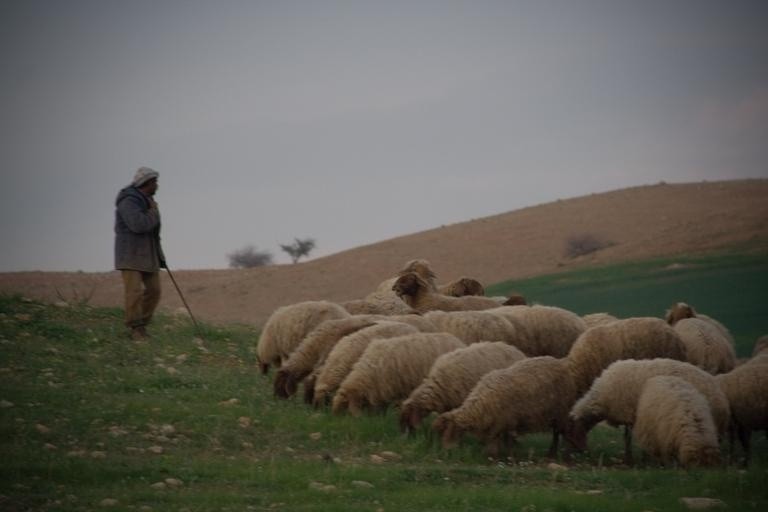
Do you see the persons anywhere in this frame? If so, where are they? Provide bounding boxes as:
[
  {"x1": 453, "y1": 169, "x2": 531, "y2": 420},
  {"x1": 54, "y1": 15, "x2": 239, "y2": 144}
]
[{"x1": 113, "y1": 165, "x2": 169, "y2": 343}]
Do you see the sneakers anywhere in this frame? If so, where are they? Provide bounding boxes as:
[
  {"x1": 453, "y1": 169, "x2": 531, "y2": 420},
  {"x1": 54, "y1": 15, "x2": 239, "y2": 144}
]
[{"x1": 130, "y1": 327, "x2": 154, "y2": 341}]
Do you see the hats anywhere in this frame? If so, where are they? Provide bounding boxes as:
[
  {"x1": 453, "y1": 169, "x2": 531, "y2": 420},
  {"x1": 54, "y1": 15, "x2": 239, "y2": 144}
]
[{"x1": 132, "y1": 167, "x2": 158, "y2": 188}]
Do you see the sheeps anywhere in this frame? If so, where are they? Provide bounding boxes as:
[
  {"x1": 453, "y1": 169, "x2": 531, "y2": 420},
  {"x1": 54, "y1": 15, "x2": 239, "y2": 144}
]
[{"x1": 255, "y1": 257, "x2": 768, "y2": 471}]
